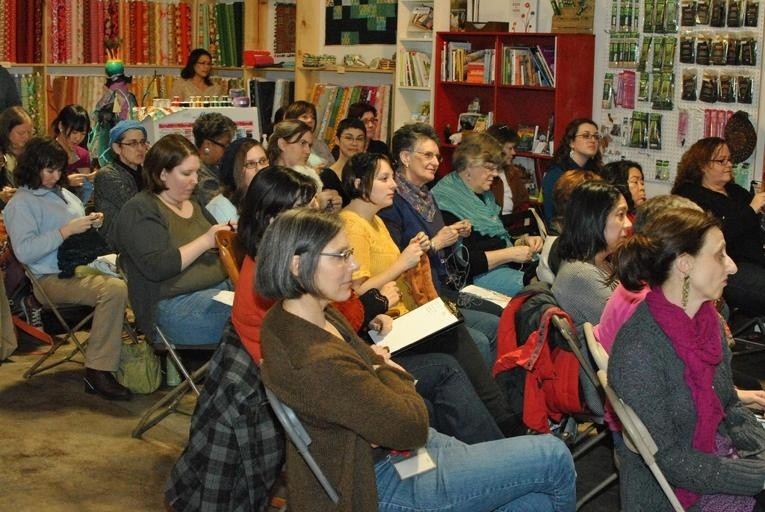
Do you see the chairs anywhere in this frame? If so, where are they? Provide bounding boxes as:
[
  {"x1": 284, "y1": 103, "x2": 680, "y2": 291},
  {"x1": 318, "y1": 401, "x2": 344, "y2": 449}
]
[
  {"x1": 5, "y1": 229, "x2": 344, "y2": 507},
  {"x1": 552, "y1": 315, "x2": 684, "y2": 511}
]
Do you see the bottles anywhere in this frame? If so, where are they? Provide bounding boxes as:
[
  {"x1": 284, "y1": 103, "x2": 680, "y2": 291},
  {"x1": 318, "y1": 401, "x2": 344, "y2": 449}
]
[
  {"x1": 171, "y1": 95, "x2": 231, "y2": 107},
  {"x1": 468, "y1": 96, "x2": 481, "y2": 113}
]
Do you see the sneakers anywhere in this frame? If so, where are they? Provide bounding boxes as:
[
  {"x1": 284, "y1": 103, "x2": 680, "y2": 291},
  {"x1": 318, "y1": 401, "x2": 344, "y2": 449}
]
[
  {"x1": 20, "y1": 295, "x2": 44, "y2": 333},
  {"x1": 84, "y1": 372, "x2": 133, "y2": 402}
]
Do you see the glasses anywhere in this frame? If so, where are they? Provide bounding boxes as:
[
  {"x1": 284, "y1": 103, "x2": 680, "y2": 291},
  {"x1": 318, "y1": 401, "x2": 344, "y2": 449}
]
[
  {"x1": 406, "y1": 150, "x2": 444, "y2": 162},
  {"x1": 241, "y1": 157, "x2": 269, "y2": 169},
  {"x1": 318, "y1": 246, "x2": 355, "y2": 265},
  {"x1": 572, "y1": 133, "x2": 603, "y2": 140},
  {"x1": 205, "y1": 136, "x2": 230, "y2": 151},
  {"x1": 119, "y1": 140, "x2": 148, "y2": 147},
  {"x1": 706, "y1": 158, "x2": 736, "y2": 166}
]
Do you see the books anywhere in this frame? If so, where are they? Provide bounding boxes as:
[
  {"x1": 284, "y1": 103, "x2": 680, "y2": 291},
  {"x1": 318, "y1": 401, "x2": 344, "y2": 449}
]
[{"x1": 438, "y1": 39, "x2": 555, "y2": 84}]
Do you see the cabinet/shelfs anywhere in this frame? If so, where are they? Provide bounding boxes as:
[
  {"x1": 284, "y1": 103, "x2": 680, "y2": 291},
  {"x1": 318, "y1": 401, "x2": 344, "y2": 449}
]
[
  {"x1": 391, "y1": 0, "x2": 452, "y2": 151},
  {"x1": 434, "y1": 29, "x2": 595, "y2": 202}
]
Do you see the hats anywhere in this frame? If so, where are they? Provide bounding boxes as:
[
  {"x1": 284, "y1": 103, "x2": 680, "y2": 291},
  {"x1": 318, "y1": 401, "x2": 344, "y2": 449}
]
[{"x1": 107, "y1": 119, "x2": 147, "y2": 148}]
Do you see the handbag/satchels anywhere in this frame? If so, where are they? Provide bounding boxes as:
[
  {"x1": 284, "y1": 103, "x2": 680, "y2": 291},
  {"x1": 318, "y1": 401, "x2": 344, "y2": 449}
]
[{"x1": 113, "y1": 322, "x2": 163, "y2": 395}]
